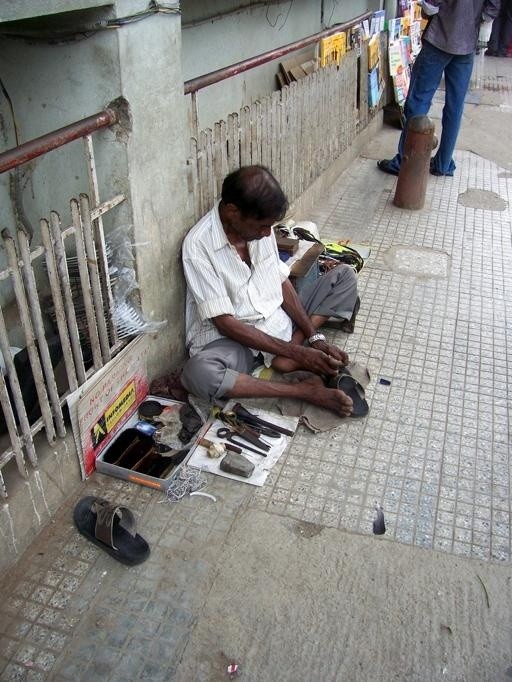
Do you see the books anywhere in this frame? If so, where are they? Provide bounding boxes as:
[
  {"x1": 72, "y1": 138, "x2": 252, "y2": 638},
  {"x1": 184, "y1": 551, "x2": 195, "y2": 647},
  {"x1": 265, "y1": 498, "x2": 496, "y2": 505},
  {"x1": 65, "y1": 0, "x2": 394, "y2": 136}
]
[
  {"x1": 368, "y1": 34, "x2": 379, "y2": 70},
  {"x1": 347, "y1": 10, "x2": 386, "y2": 49},
  {"x1": 320, "y1": 32, "x2": 347, "y2": 66},
  {"x1": 368, "y1": 65, "x2": 379, "y2": 107},
  {"x1": 388, "y1": 0, "x2": 428, "y2": 114}
]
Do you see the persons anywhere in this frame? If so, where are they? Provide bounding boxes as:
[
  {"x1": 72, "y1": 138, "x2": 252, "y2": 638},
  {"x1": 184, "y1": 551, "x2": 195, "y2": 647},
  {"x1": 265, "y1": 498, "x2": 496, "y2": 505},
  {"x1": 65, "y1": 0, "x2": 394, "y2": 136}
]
[
  {"x1": 377, "y1": 0, "x2": 502, "y2": 177},
  {"x1": 180, "y1": 163, "x2": 358, "y2": 419}
]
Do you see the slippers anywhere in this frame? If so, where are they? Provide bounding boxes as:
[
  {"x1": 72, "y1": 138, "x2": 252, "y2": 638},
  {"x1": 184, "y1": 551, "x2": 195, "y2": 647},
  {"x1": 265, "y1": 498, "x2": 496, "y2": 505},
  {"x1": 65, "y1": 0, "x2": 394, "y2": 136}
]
[
  {"x1": 326, "y1": 362, "x2": 369, "y2": 418},
  {"x1": 376, "y1": 157, "x2": 406, "y2": 175},
  {"x1": 73, "y1": 493, "x2": 151, "y2": 566}
]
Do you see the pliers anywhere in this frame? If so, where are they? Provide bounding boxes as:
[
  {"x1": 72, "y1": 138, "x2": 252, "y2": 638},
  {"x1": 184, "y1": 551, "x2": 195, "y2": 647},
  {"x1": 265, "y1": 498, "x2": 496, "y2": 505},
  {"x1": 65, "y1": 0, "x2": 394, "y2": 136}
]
[
  {"x1": 217, "y1": 427, "x2": 267, "y2": 457},
  {"x1": 242, "y1": 422, "x2": 281, "y2": 438}
]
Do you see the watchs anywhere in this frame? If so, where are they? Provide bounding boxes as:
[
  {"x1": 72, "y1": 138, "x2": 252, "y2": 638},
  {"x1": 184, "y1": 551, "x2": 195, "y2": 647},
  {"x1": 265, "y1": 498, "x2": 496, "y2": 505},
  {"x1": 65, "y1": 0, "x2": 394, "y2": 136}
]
[{"x1": 309, "y1": 334, "x2": 326, "y2": 344}]
